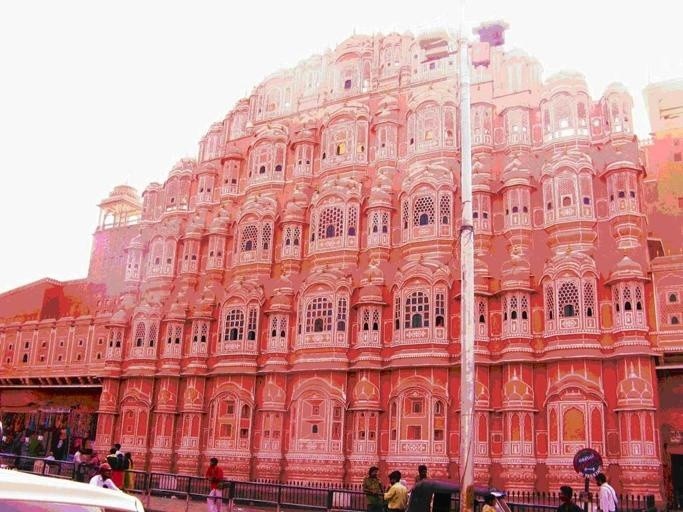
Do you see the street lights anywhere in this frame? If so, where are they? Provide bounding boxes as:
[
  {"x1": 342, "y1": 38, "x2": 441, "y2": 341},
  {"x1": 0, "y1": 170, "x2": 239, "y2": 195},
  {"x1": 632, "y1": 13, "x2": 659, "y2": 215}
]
[{"x1": 417, "y1": 13, "x2": 492, "y2": 512}]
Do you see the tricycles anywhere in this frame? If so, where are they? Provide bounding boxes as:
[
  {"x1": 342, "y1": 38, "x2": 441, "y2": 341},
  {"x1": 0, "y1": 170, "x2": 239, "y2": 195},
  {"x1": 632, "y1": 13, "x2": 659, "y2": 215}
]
[{"x1": 404, "y1": 479, "x2": 512, "y2": 512}]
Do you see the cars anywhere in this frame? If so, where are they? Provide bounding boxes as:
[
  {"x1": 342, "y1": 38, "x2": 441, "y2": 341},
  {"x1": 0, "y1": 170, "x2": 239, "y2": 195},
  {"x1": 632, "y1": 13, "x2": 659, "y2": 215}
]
[{"x1": 0, "y1": 467, "x2": 145, "y2": 511}]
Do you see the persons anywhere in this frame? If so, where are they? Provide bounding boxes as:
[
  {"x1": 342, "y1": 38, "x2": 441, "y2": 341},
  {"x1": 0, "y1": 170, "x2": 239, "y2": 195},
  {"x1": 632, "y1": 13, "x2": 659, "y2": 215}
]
[
  {"x1": 0, "y1": 419, "x2": 136, "y2": 493},
  {"x1": 361, "y1": 465, "x2": 433, "y2": 510},
  {"x1": 204, "y1": 457, "x2": 223, "y2": 512},
  {"x1": 594, "y1": 472, "x2": 618, "y2": 511},
  {"x1": 555, "y1": 483, "x2": 585, "y2": 511},
  {"x1": 480, "y1": 494, "x2": 500, "y2": 512}
]
